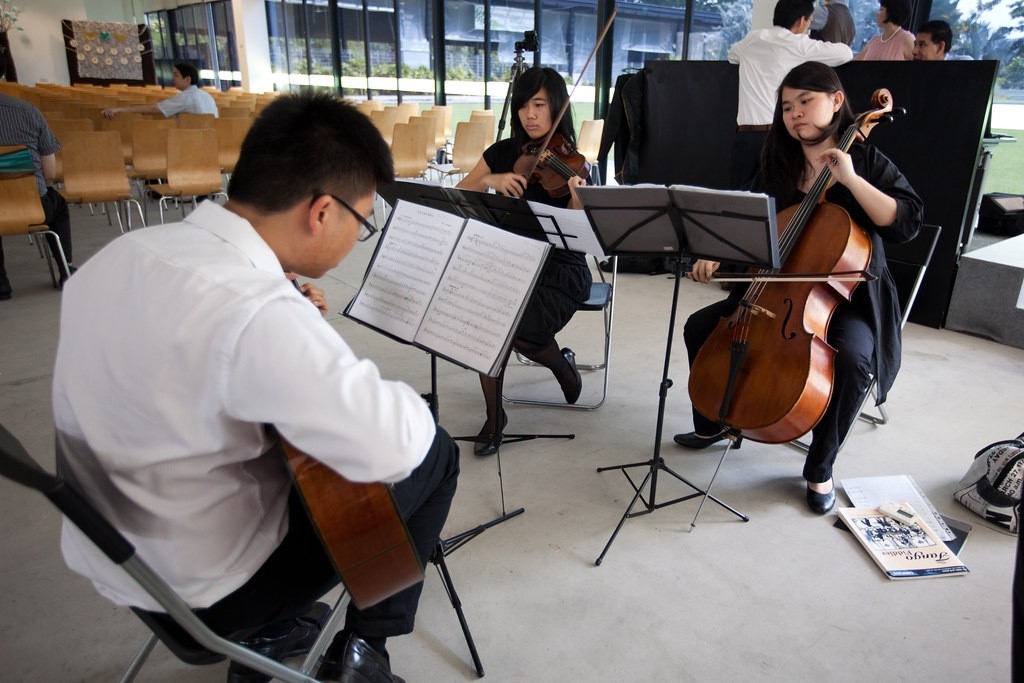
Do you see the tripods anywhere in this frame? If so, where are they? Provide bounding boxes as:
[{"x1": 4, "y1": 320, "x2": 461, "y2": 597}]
[
  {"x1": 495, "y1": 42, "x2": 531, "y2": 144},
  {"x1": 346, "y1": 177, "x2": 772, "y2": 679}
]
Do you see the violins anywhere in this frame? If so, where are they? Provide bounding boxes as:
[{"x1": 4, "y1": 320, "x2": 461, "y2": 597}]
[{"x1": 513, "y1": 135, "x2": 592, "y2": 199}]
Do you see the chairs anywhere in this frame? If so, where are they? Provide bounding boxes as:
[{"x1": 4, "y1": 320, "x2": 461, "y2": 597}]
[
  {"x1": 779, "y1": 221, "x2": 942, "y2": 454},
  {"x1": 1, "y1": 426, "x2": 366, "y2": 683},
  {"x1": 492, "y1": 240, "x2": 620, "y2": 417},
  {"x1": 0, "y1": 82, "x2": 502, "y2": 295},
  {"x1": 570, "y1": 115, "x2": 604, "y2": 187}
]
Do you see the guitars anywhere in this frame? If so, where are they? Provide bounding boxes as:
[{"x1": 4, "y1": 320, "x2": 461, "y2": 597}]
[{"x1": 268, "y1": 268, "x2": 427, "y2": 610}]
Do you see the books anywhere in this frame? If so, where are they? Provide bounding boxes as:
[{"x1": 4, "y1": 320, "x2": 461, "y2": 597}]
[{"x1": 836, "y1": 501, "x2": 970, "y2": 581}]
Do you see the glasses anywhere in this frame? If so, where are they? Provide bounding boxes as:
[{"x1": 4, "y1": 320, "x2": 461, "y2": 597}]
[{"x1": 334, "y1": 197, "x2": 378, "y2": 241}]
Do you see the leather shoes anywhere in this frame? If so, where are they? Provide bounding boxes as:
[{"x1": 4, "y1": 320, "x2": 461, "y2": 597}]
[
  {"x1": 315, "y1": 630, "x2": 408, "y2": 683},
  {"x1": 227, "y1": 601, "x2": 332, "y2": 683}
]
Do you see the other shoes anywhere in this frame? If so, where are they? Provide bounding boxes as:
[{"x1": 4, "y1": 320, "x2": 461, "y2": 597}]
[
  {"x1": 59, "y1": 268, "x2": 78, "y2": 291},
  {"x1": 147, "y1": 191, "x2": 162, "y2": 199}
]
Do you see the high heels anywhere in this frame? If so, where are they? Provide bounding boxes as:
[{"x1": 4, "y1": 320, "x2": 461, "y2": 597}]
[
  {"x1": 674, "y1": 428, "x2": 743, "y2": 450},
  {"x1": 805, "y1": 475, "x2": 836, "y2": 513},
  {"x1": 473, "y1": 407, "x2": 507, "y2": 456},
  {"x1": 559, "y1": 347, "x2": 583, "y2": 406}
]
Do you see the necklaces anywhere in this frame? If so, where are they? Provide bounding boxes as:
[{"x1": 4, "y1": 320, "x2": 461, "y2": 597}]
[{"x1": 880, "y1": 26, "x2": 901, "y2": 43}]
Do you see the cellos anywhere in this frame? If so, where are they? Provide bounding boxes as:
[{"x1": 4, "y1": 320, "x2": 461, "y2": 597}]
[{"x1": 689, "y1": 88, "x2": 907, "y2": 528}]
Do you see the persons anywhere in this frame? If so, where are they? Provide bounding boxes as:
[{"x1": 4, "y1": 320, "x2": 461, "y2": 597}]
[
  {"x1": 459, "y1": 65, "x2": 592, "y2": 456},
  {"x1": 51, "y1": 91, "x2": 460, "y2": 683},
  {"x1": 673, "y1": 60, "x2": 925, "y2": 515},
  {"x1": 729, "y1": 0, "x2": 975, "y2": 191},
  {"x1": 101, "y1": 63, "x2": 218, "y2": 197},
  {"x1": 0, "y1": 93, "x2": 77, "y2": 301}
]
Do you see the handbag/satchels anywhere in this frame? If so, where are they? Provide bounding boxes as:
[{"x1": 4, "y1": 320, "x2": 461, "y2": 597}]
[{"x1": 952, "y1": 431, "x2": 1024, "y2": 533}]
